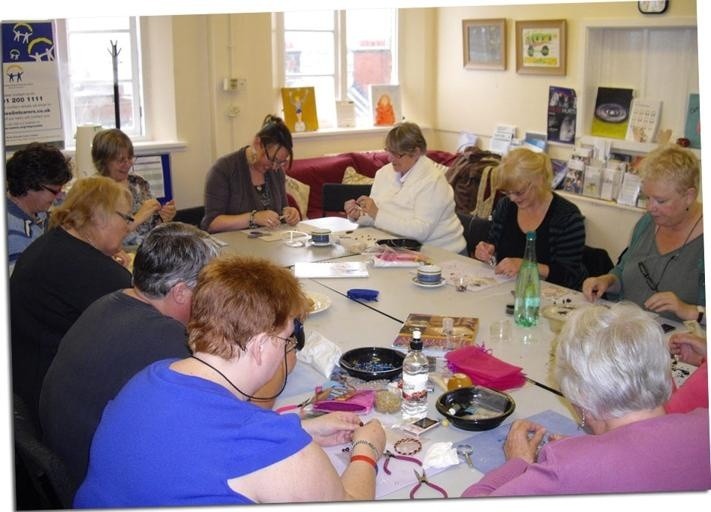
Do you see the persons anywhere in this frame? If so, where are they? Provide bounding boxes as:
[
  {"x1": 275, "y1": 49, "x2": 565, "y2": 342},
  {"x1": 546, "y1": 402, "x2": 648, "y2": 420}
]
[
  {"x1": 344, "y1": 122, "x2": 469, "y2": 257},
  {"x1": 474, "y1": 148, "x2": 586, "y2": 290},
  {"x1": 38, "y1": 220, "x2": 221, "y2": 487},
  {"x1": 460, "y1": 299, "x2": 711, "y2": 497},
  {"x1": 70, "y1": 256, "x2": 386, "y2": 509},
  {"x1": 9, "y1": 176, "x2": 133, "y2": 431},
  {"x1": 200, "y1": 116, "x2": 300, "y2": 234},
  {"x1": 91, "y1": 128, "x2": 177, "y2": 252},
  {"x1": 659, "y1": 333, "x2": 708, "y2": 414},
  {"x1": 582, "y1": 145, "x2": 706, "y2": 328},
  {"x1": 6, "y1": 142, "x2": 72, "y2": 280}
]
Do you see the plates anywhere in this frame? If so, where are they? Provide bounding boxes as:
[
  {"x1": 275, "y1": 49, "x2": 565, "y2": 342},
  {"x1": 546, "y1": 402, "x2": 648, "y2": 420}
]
[
  {"x1": 409, "y1": 274, "x2": 445, "y2": 288},
  {"x1": 541, "y1": 303, "x2": 580, "y2": 322},
  {"x1": 304, "y1": 291, "x2": 332, "y2": 314},
  {"x1": 307, "y1": 239, "x2": 332, "y2": 247}
]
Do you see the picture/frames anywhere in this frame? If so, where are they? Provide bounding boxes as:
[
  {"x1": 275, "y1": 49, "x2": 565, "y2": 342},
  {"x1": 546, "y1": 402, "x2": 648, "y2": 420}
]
[
  {"x1": 460, "y1": 17, "x2": 509, "y2": 70},
  {"x1": 638, "y1": 0, "x2": 671, "y2": 15},
  {"x1": 514, "y1": 16, "x2": 567, "y2": 78}
]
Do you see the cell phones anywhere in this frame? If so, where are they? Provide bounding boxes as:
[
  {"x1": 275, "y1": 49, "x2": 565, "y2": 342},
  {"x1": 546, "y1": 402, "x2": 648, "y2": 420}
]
[{"x1": 404, "y1": 417, "x2": 440, "y2": 435}]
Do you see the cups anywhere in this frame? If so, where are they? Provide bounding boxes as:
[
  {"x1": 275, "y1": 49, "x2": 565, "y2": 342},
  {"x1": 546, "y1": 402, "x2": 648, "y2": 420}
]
[
  {"x1": 312, "y1": 228, "x2": 332, "y2": 243},
  {"x1": 416, "y1": 265, "x2": 441, "y2": 284},
  {"x1": 490, "y1": 318, "x2": 513, "y2": 344},
  {"x1": 454, "y1": 277, "x2": 469, "y2": 293},
  {"x1": 374, "y1": 385, "x2": 403, "y2": 413}
]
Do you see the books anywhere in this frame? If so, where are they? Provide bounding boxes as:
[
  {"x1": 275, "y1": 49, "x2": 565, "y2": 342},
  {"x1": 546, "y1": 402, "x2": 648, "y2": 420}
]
[
  {"x1": 393, "y1": 313, "x2": 480, "y2": 351},
  {"x1": 370, "y1": 84, "x2": 403, "y2": 128},
  {"x1": 454, "y1": 83, "x2": 700, "y2": 210},
  {"x1": 281, "y1": 87, "x2": 318, "y2": 133}
]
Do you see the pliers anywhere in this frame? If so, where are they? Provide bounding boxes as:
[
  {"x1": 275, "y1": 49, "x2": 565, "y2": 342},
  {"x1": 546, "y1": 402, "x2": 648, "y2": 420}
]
[
  {"x1": 382, "y1": 449, "x2": 422, "y2": 475},
  {"x1": 247, "y1": 231, "x2": 270, "y2": 238},
  {"x1": 276, "y1": 398, "x2": 313, "y2": 418},
  {"x1": 410, "y1": 468, "x2": 448, "y2": 499}
]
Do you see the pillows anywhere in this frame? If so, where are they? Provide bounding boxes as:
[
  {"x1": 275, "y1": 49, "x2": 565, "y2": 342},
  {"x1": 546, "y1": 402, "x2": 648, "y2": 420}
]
[
  {"x1": 340, "y1": 165, "x2": 379, "y2": 214},
  {"x1": 285, "y1": 173, "x2": 314, "y2": 217}
]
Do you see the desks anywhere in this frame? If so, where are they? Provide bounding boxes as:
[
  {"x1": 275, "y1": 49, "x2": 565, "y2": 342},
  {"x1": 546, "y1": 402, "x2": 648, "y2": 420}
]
[{"x1": 109, "y1": 226, "x2": 705, "y2": 500}]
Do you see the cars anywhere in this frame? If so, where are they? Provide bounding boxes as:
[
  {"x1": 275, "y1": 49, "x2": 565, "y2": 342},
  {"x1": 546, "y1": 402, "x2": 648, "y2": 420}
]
[{"x1": 73, "y1": 91, "x2": 143, "y2": 143}]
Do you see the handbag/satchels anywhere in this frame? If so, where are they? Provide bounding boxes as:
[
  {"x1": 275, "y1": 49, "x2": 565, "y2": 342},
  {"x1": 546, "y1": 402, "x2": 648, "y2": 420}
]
[{"x1": 313, "y1": 386, "x2": 375, "y2": 415}]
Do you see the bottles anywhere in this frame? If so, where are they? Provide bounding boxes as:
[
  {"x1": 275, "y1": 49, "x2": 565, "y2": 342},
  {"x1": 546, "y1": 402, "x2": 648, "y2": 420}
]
[
  {"x1": 512, "y1": 230, "x2": 542, "y2": 328},
  {"x1": 401, "y1": 329, "x2": 431, "y2": 422},
  {"x1": 433, "y1": 316, "x2": 456, "y2": 380}
]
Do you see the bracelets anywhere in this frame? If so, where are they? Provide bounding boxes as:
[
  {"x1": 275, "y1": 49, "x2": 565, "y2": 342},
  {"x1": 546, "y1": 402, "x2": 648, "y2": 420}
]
[
  {"x1": 696, "y1": 304, "x2": 704, "y2": 323},
  {"x1": 249, "y1": 210, "x2": 257, "y2": 229},
  {"x1": 350, "y1": 455, "x2": 378, "y2": 474},
  {"x1": 352, "y1": 440, "x2": 380, "y2": 461},
  {"x1": 394, "y1": 438, "x2": 422, "y2": 455}
]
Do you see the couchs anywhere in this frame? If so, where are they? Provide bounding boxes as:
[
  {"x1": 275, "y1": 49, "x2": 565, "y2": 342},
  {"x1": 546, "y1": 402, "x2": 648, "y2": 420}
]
[{"x1": 283, "y1": 149, "x2": 496, "y2": 222}]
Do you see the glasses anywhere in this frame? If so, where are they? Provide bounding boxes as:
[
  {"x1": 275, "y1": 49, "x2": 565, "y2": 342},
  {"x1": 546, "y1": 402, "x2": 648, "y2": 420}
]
[
  {"x1": 638, "y1": 255, "x2": 676, "y2": 292},
  {"x1": 262, "y1": 144, "x2": 288, "y2": 167},
  {"x1": 270, "y1": 335, "x2": 297, "y2": 353},
  {"x1": 114, "y1": 210, "x2": 134, "y2": 225},
  {"x1": 498, "y1": 182, "x2": 531, "y2": 196},
  {"x1": 383, "y1": 147, "x2": 406, "y2": 159},
  {"x1": 41, "y1": 184, "x2": 61, "y2": 196},
  {"x1": 115, "y1": 156, "x2": 138, "y2": 167}
]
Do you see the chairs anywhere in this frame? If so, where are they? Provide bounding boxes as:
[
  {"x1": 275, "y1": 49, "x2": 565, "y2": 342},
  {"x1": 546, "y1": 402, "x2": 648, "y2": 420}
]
[{"x1": 319, "y1": 179, "x2": 376, "y2": 218}]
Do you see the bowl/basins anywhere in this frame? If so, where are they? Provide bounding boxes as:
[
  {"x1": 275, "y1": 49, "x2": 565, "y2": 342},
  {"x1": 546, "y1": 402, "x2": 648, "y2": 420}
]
[
  {"x1": 338, "y1": 346, "x2": 407, "y2": 382},
  {"x1": 436, "y1": 386, "x2": 523, "y2": 431}
]
